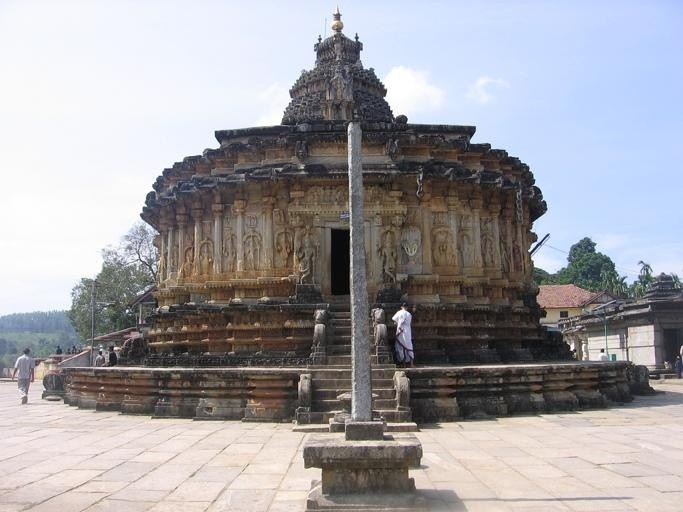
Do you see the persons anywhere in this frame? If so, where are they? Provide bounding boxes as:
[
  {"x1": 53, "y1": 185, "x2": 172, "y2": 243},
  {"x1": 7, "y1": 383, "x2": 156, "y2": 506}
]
[
  {"x1": 599, "y1": 347, "x2": 608, "y2": 361},
  {"x1": 11, "y1": 348, "x2": 36, "y2": 406},
  {"x1": 390, "y1": 301, "x2": 416, "y2": 367},
  {"x1": 94, "y1": 350, "x2": 105, "y2": 366},
  {"x1": 679, "y1": 344, "x2": 683, "y2": 363},
  {"x1": 54, "y1": 343, "x2": 82, "y2": 355},
  {"x1": 571, "y1": 348, "x2": 579, "y2": 359},
  {"x1": 673, "y1": 354, "x2": 682, "y2": 377},
  {"x1": 105, "y1": 346, "x2": 117, "y2": 366}
]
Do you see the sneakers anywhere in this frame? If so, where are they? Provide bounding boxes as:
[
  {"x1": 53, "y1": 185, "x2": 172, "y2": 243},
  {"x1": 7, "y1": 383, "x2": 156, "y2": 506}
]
[{"x1": 21, "y1": 396, "x2": 28, "y2": 405}]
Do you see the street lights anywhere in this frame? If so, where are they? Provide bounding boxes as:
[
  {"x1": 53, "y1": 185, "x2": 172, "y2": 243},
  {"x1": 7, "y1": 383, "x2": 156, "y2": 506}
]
[{"x1": 582, "y1": 299, "x2": 617, "y2": 361}]
